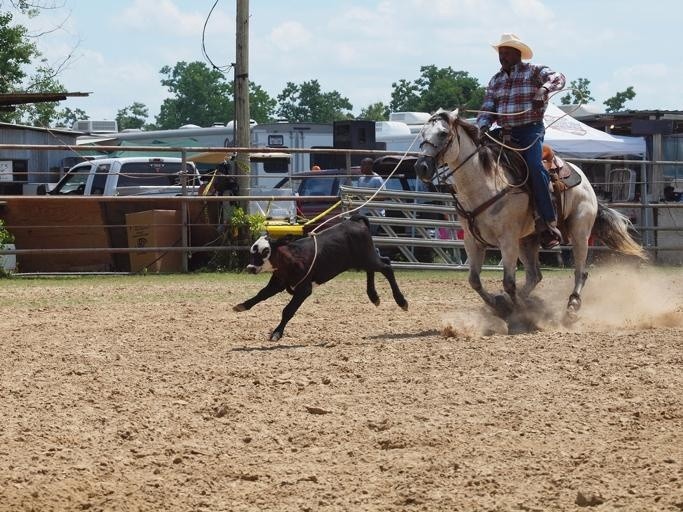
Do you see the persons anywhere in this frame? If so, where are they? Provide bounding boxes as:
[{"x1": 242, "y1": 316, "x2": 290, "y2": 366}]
[
  {"x1": 661, "y1": 185, "x2": 681, "y2": 203},
  {"x1": 356, "y1": 157, "x2": 387, "y2": 234},
  {"x1": 473, "y1": 33, "x2": 566, "y2": 248}
]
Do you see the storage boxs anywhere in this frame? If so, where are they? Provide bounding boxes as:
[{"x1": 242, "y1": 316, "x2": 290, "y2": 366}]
[{"x1": 122, "y1": 208, "x2": 184, "y2": 275}]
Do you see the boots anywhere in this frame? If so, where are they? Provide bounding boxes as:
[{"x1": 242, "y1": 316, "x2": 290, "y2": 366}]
[{"x1": 539, "y1": 219, "x2": 559, "y2": 249}]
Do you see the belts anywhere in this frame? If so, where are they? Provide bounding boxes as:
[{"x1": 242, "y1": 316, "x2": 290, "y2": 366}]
[{"x1": 503, "y1": 121, "x2": 540, "y2": 129}]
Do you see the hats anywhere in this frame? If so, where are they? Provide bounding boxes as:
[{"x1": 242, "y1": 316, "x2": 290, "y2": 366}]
[
  {"x1": 489, "y1": 32, "x2": 534, "y2": 60},
  {"x1": 312, "y1": 166, "x2": 320, "y2": 172}
]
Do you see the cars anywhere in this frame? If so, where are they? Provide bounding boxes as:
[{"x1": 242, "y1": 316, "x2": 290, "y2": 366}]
[{"x1": 271, "y1": 168, "x2": 448, "y2": 264}]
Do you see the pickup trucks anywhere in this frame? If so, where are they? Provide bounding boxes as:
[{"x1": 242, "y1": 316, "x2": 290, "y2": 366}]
[{"x1": 36, "y1": 157, "x2": 297, "y2": 265}]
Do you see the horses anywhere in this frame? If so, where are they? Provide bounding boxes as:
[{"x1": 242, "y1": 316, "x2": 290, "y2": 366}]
[{"x1": 414, "y1": 107, "x2": 650, "y2": 335}]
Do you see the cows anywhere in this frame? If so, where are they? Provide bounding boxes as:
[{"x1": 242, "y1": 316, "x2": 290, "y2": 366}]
[{"x1": 233, "y1": 214, "x2": 408, "y2": 341}]
